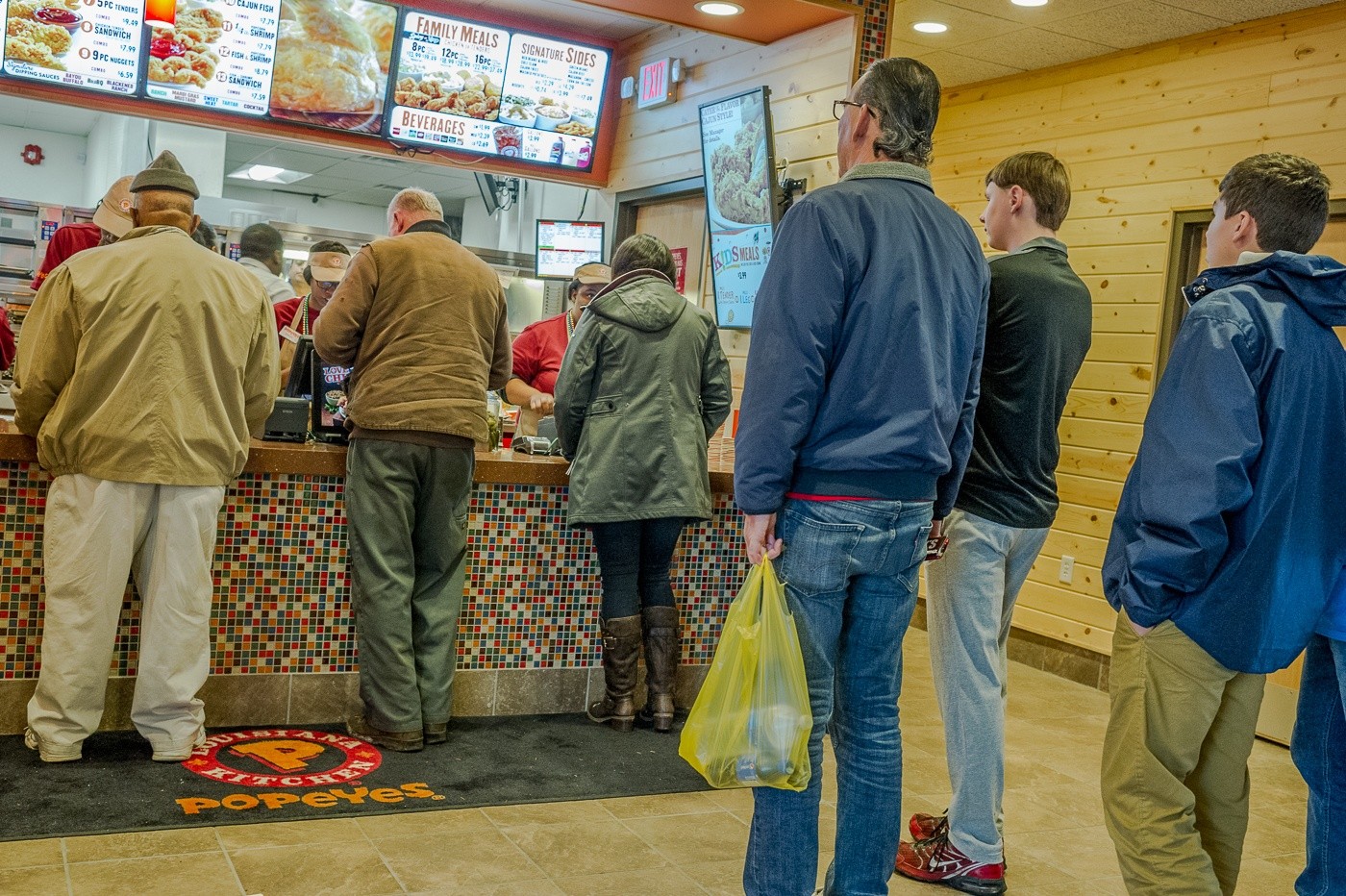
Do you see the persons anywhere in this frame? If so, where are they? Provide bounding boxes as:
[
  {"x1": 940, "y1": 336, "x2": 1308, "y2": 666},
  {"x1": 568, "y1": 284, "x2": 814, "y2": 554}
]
[
  {"x1": 235, "y1": 222, "x2": 297, "y2": 308},
  {"x1": 1288, "y1": 558, "x2": 1346, "y2": 896},
  {"x1": 288, "y1": 256, "x2": 313, "y2": 298},
  {"x1": 553, "y1": 230, "x2": 734, "y2": 734},
  {"x1": 728, "y1": 56, "x2": 989, "y2": 896},
  {"x1": 310, "y1": 188, "x2": 514, "y2": 756},
  {"x1": 8, "y1": 151, "x2": 283, "y2": 768},
  {"x1": 1095, "y1": 150, "x2": 1345, "y2": 895},
  {"x1": 28, "y1": 174, "x2": 136, "y2": 291},
  {"x1": 895, "y1": 151, "x2": 1092, "y2": 896},
  {"x1": 188, "y1": 218, "x2": 222, "y2": 254},
  {"x1": 272, "y1": 239, "x2": 353, "y2": 398},
  {"x1": 494, "y1": 260, "x2": 612, "y2": 447}
]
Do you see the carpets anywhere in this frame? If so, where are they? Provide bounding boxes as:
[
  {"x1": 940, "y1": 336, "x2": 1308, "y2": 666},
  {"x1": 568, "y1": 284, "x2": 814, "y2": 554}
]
[{"x1": 0, "y1": 705, "x2": 719, "y2": 843}]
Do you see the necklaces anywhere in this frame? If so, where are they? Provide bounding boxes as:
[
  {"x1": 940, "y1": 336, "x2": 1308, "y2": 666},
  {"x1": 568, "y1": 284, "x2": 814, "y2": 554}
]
[
  {"x1": 302, "y1": 293, "x2": 311, "y2": 336},
  {"x1": 567, "y1": 309, "x2": 575, "y2": 338}
]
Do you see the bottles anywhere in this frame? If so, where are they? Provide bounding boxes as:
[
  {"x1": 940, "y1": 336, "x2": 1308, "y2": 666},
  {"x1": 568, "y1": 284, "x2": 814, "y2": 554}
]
[
  {"x1": 693, "y1": 698, "x2": 812, "y2": 785},
  {"x1": 562, "y1": 137, "x2": 578, "y2": 165},
  {"x1": 576, "y1": 140, "x2": 593, "y2": 167},
  {"x1": 548, "y1": 137, "x2": 566, "y2": 163}
]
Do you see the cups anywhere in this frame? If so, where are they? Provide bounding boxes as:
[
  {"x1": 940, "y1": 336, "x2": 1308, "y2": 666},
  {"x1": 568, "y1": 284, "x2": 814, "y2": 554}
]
[
  {"x1": 535, "y1": 104, "x2": 571, "y2": 132},
  {"x1": 499, "y1": 106, "x2": 536, "y2": 128},
  {"x1": 570, "y1": 108, "x2": 597, "y2": 127}
]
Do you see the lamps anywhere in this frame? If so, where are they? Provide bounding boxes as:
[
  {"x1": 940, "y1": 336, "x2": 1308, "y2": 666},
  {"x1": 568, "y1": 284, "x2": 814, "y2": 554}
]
[{"x1": 621, "y1": 56, "x2": 686, "y2": 109}]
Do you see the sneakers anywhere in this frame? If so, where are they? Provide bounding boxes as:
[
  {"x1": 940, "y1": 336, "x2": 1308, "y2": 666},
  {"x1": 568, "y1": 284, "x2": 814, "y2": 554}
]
[
  {"x1": 894, "y1": 812, "x2": 1007, "y2": 896},
  {"x1": 152, "y1": 723, "x2": 206, "y2": 761},
  {"x1": 345, "y1": 707, "x2": 447, "y2": 752},
  {"x1": 23, "y1": 726, "x2": 83, "y2": 763}
]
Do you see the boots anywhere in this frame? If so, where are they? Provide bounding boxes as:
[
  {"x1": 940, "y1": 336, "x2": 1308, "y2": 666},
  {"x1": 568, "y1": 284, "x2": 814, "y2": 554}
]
[{"x1": 587, "y1": 605, "x2": 679, "y2": 732}]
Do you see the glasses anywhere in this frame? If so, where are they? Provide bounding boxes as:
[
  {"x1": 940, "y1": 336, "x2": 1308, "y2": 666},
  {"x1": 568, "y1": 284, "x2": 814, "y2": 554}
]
[
  {"x1": 315, "y1": 279, "x2": 340, "y2": 290},
  {"x1": 832, "y1": 100, "x2": 877, "y2": 121}
]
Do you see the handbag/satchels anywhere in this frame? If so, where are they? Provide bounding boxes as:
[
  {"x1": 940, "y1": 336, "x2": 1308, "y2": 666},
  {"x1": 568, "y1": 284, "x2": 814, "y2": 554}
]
[{"x1": 678, "y1": 552, "x2": 814, "y2": 792}]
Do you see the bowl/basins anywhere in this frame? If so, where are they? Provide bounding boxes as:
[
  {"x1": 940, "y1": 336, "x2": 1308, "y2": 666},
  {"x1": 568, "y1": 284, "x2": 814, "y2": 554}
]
[{"x1": 325, "y1": 392, "x2": 343, "y2": 405}]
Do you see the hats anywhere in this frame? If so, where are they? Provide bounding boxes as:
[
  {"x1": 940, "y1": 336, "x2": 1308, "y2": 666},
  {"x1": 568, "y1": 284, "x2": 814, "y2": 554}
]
[
  {"x1": 92, "y1": 176, "x2": 137, "y2": 239},
  {"x1": 572, "y1": 264, "x2": 612, "y2": 284},
  {"x1": 129, "y1": 150, "x2": 200, "y2": 201},
  {"x1": 307, "y1": 251, "x2": 351, "y2": 282}
]
